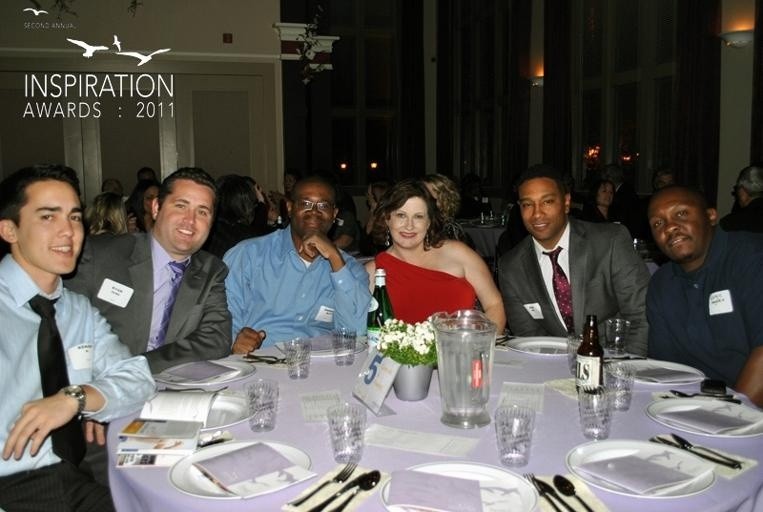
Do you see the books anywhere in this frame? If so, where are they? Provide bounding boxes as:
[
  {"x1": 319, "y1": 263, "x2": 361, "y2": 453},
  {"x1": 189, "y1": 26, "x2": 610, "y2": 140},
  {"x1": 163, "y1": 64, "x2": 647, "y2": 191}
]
[{"x1": 115, "y1": 387, "x2": 229, "y2": 468}]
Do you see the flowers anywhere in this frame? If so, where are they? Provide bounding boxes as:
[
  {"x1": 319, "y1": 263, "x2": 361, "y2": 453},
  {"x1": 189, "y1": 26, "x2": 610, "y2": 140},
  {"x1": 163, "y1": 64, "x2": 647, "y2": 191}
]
[{"x1": 379, "y1": 318, "x2": 438, "y2": 363}]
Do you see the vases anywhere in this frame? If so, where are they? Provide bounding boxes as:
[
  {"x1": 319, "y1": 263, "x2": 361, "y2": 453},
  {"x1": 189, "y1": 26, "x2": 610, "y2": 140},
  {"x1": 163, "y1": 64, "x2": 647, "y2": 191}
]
[{"x1": 390, "y1": 355, "x2": 433, "y2": 401}]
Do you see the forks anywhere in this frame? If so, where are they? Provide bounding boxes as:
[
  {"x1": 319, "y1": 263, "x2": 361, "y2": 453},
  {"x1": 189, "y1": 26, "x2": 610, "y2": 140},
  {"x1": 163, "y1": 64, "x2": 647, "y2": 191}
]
[
  {"x1": 522, "y1": 472, "x2": 563, "y2": 512},
  {"x1": 287, "y1": 463, "x2": 357, "y2": 507}
]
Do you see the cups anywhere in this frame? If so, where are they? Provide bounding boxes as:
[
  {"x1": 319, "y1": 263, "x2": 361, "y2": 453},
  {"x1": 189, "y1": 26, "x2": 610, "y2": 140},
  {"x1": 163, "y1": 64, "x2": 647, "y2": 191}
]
[
  {"x1": 575, "y1": 318, "x2": 636, "y2": 440},
  {"x1": 248, "y1": 310, "x2": 537, "y2": 469}
]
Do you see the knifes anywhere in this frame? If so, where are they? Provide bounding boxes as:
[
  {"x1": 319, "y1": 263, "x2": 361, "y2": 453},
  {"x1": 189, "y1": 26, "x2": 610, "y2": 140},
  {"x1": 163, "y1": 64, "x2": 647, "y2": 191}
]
[
  {"x1": 309, "y1": 473, "x2": 367, "y2": 512},
  {"x1": 534, "y1": 478, "x2": 576, "y2": 512},
  {"x1": 657, "y1": 437, "x2": 738, "y2": 469}
]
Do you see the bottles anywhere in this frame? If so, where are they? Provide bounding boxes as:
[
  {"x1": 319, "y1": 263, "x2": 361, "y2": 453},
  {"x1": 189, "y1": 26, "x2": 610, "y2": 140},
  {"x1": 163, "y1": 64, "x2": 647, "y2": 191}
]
[
  {"x1": 577, "y1": 315, "x2": 604, "y2": 394},
  {"x1": 366, "y1": 268, "x2": 394, "y2": 355}
]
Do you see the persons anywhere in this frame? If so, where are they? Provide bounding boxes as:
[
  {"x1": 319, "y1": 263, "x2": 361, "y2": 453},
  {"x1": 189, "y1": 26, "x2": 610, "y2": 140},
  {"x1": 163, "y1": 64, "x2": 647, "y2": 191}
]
[
  {"x1": 498, "y1": 165, "x2": 651, "y2": 359},
  {"x1": 63, "y1": 168, "x2": 234, "y2": 379},
  {"x1": 645, "y1": 185, "x2": 762, "y2": 408},
  {"x1": 364, "y1": 179, "x2": 508, "y2": 337},
  {"x1": 81, "y1": 160, "x2": 763, "y2": 259},
  {"x1": 0, "y1": 163, "x2": 156, "y2": 512},
  {"x1": 222, "y1": 176, "x2": 371, "y2": 360}
]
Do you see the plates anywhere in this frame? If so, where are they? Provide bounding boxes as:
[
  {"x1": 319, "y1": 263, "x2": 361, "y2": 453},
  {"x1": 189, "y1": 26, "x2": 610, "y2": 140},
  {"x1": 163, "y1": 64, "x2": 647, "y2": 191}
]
[
  {"x1": 505, "y1": 336, "x2": 567, "y2": 356},
  {"x1": 379, "y1": 461, "x2": 539, "y2": 512},
  {"x1": 611, "y1": 360, "x2": 704, "y2": 386},
  {"x1": 645, "y1": 398, "x2": 763, "y2": 438},
  {"x1": 159, "y1": 359, "x2": 256, "y2": 385},
  {"x1": 167, "y1": 440, "x2": 312, "y2": 500},
  {"x1": 201, "y1": 394, "x2": 247, "y2": 432},
  {"x1": 566, "y1": 439, "x2": 715, "y2": 499}
]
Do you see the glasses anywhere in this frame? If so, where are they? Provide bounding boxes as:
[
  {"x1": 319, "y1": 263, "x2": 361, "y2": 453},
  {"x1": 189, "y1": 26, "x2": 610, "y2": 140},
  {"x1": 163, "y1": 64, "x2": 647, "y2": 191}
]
[{"x1": 292, "y1": 199, "x2": 335, "y2": 212}]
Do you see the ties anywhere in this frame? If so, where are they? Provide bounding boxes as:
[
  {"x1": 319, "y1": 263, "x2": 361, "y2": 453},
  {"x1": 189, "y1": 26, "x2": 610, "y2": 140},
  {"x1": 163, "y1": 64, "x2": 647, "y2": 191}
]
[
  {"x1": 543, "y1": 246, "x2": 576, "y2": 336},
  {"x1": 28, "y1": 295, "x2": 85, "y2": 470},
  {"x1": 155, "y1": 260, "x2": 189, "y2": 348}
]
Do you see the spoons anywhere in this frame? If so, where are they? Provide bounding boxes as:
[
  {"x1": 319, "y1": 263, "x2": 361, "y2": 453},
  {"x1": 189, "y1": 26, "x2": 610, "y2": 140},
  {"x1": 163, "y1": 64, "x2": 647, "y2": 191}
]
[
  {"x1": 554, "y1": 475, "x2": 596, "y2": 512},
  {"x1": 669, "y1": 390, "x2": 734, "y2": 399},
  {"x1": 671, "y1": 433, "x2": 745, "y2": 464},
  {"x1": 331, "y1": 471, "x2": 379, "y2": 512}
]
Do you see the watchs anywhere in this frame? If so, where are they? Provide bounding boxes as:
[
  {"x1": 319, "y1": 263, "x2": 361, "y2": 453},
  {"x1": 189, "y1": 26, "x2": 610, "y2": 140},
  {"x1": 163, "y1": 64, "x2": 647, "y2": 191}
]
[{"x1": 56, "y1": 386, "x2": 86, "y2": 421}]
